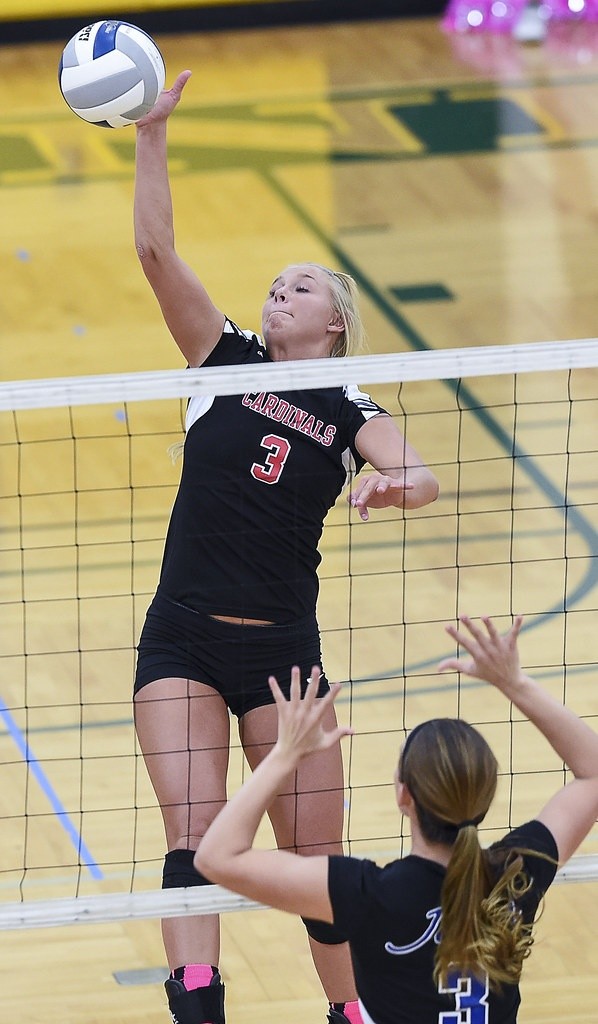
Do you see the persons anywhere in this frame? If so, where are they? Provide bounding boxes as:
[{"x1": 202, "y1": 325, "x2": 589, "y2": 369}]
[
  {"x1": 194, "y1": 613, "x2": 598, "y2": 1024},
  {"x1": 134, "y1": 70, "x2": 441, "y2": 1024}
]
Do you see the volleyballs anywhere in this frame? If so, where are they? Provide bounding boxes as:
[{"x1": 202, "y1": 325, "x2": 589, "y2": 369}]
[{"x1": 58, "y1": 20, "x2": 167, "y2": 129}]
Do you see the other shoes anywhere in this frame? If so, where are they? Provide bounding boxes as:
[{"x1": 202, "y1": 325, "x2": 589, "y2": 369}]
[
  {"x1": 164, "y1": 974, "x2": 228, "y2": 1023},
  {"x1": 326, "y1": 1009, "x2": 352, "y2": 1024}
]
